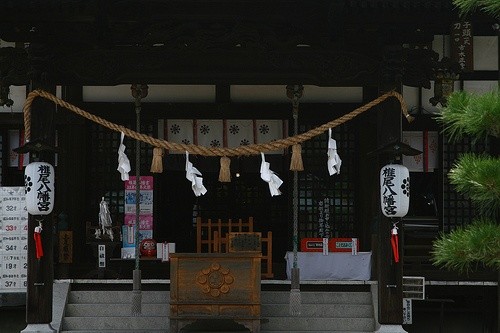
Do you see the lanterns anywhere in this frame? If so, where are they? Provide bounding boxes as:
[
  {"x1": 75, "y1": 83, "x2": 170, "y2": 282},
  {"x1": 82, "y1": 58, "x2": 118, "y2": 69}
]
[
  {"x1": 380, "y1": 164, "x2": 410, "y2": 217},
  {"x1": 24, "y1": 161, "x2": 55, "y2": 216}
]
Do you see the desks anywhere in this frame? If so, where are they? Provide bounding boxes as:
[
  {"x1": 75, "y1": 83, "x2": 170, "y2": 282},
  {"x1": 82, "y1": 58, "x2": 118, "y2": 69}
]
[
  {"x1": 109, "y1": 256, "x2": 169, "y2": 279},
  {"x1": 285, "y1": 251, "x2": 371, "y2": 280}
]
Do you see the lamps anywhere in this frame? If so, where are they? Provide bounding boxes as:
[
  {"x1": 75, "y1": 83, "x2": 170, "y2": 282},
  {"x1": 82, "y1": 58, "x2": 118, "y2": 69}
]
[{"x1": 429, "y1": 28, "x2": 472, "y2": 109}]
